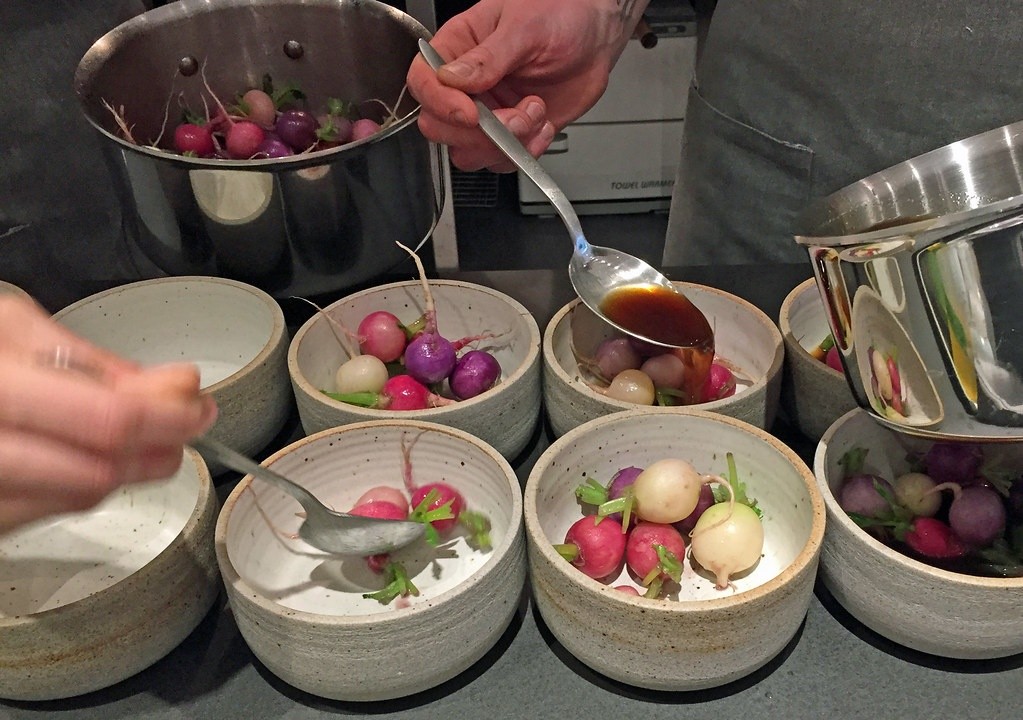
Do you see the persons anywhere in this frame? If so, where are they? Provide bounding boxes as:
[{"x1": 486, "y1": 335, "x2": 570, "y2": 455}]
[
  {"x1": 0, "y1": 0, "x2": 436, "y2": 536},
  {"x1": 407, "y1": 0, "x2": 1023, "y2": 383}
]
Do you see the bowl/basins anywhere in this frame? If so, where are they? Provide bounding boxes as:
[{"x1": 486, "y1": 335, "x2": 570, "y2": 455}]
[
  {"x1": 541, "y1": 279, "x2": 785, "y2": 441},
  {"x1": 286, "y1": 278, "x2": 542, "y2": 463},
  {"x1": 72, "y1": 0, "x2": 447, "y2": 310},
  {"x1": 48, "y1": 274, "x2": 294, "y2": 479},
  {"x1": 523, "y1": 409, "x2": 827, "y2": 692},
  {"x1": 812, "y1": 408, "x2": 1023, "y2": 660},
  {"x1": 0, "y1": 446, "x2": 225, "y2": 702},
  {"x1": 778, "y1": 276, "x2": 861, "y2": 444},
  {"x1": 791, "y1": 121, "x2": 1023, "y2": 445},
  {"x1": 214, "y1": 418, "x2": 528, "y2": 702}
]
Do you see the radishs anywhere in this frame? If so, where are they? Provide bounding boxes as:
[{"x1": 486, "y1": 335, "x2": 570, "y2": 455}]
[
  {"x1": 893, "y1": 472, "x2": 942, "y2": 516},
  {"x1": 632, "y1": 458, "x2": 735, "y2": 536},
  {"x1": 691, "y1": 500, "x2": 764, "y2": 588},
  {"x1": 588, "y1": 368, "x2": 655, "y2": 407},
  {"x1": 336, "y1": 332, "x2": 390, "y2": 394}
]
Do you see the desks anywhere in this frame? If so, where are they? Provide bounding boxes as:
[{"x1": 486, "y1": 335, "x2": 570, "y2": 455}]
[{"x1": 0, "y1": 264, "x2": 1023, "y2": 719}]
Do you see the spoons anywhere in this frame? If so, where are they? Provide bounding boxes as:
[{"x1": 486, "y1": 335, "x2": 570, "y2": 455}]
[
  {"x1": 418, "y1": 39, "x2": 714, "y2": 351},
  {"x1": 188, "y1": 435, "x2": 427, "y2": 558}
]
